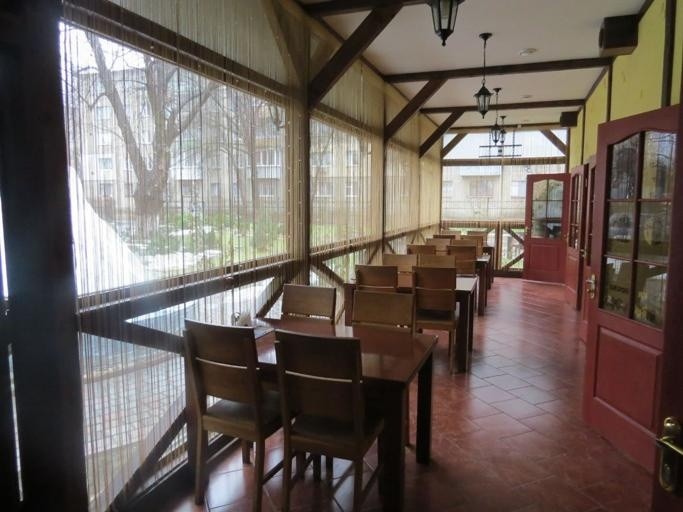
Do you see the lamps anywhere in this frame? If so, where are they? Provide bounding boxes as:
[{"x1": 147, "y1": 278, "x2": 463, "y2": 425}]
[
  {"x1": 425, "y1": 1, "x2": 465, "y2": 47},
  {"x1": 489, "y1": 88, "x2": 502, "y2": 144},
  {"x1": 497, "y1": 116, "x2": 506, "y2": 144},
  {"x1": 473, "y1": 33, "x2": 495, "y2": 120}
]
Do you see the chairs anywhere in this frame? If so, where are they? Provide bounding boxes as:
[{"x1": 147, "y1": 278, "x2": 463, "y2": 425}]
[
  {"x1": 179, "y1": 318, "x2": 306, "y2": 512},
  {"x1": 279, "y1": 282, "x2": 338, "y2": 323},
  {"x1": 340, "y1": 229, "x2": 498, "y2": 376},
  {"x1": 271, "y1": 327, "x2": 382, "y2": 510}
]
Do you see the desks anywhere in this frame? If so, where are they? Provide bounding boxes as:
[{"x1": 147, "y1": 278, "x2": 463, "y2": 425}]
[{"x1": 180, "y1": 316, "x2": 442, "y2": 476}]
